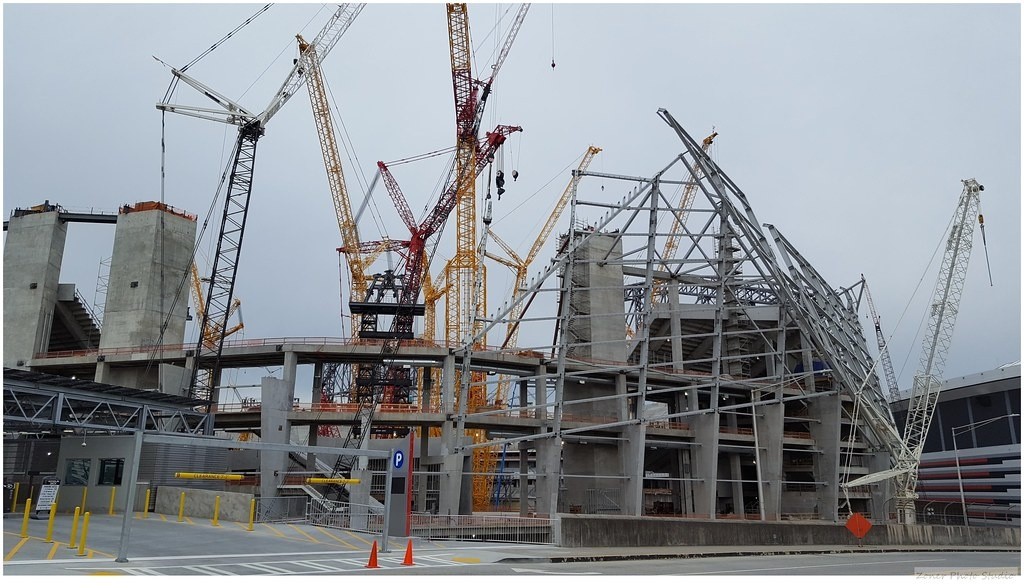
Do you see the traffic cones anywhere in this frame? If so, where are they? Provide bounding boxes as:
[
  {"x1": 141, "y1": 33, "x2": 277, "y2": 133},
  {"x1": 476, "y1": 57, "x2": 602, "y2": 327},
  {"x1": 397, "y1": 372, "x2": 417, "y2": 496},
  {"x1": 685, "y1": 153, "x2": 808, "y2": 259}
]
[
  {"x1": 400, "y1": 540, "x2": 416, "y2": 566},
  {"x1": 364, "y1": 540, "x2": 382, "y2": 568}
]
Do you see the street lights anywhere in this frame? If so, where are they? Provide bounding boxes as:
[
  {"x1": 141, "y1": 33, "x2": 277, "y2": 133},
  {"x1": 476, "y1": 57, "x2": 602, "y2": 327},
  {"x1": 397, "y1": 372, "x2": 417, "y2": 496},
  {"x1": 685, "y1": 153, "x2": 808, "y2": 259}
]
[
  {"x1": 750, "y1": 369, "x2": 834, "y2": 521},
  {"x1": 951, "y1": 413, "x2": 1019, "y2": 526}
]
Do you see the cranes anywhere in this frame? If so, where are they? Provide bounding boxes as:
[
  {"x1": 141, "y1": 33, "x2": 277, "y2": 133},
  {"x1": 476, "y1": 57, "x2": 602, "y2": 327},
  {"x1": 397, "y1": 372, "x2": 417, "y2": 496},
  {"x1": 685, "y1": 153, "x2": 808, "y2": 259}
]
[
  {"x1": 153, "y1": 2, "x2": 719, "y2": 511},
  {"x1": 840, "y1": 179, "x2": 985, "y2": 522},
  {"x1": 858, "y1": 275, "x2": 901, "y2": 401}
]
